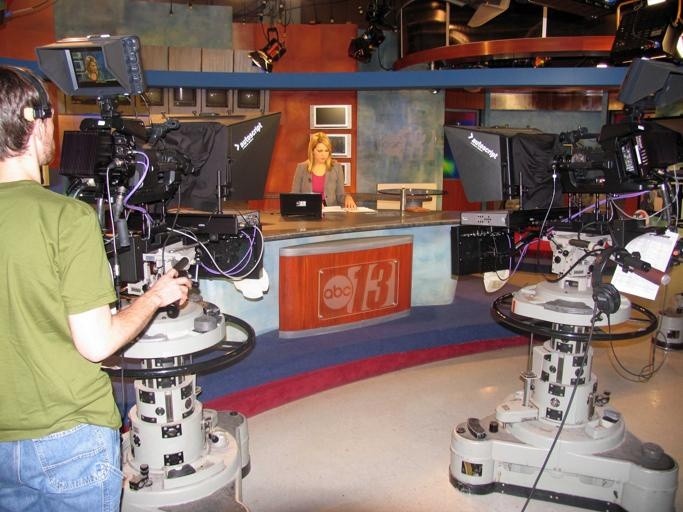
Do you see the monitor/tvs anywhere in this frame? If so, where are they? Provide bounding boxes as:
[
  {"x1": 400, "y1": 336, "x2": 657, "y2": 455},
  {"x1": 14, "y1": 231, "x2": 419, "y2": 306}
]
[
  {"x1": 279, "y1": 192, "x2": 322, "y2": 220},
  {"x1": 72, "y1": 87, "x2": 268, "y2": 114},
  {"x1": 69, "y1": 51, "x2": 121, "y2": 88}
]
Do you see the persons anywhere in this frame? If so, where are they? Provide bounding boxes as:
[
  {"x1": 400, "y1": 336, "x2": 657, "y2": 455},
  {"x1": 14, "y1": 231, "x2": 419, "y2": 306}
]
[
  {"x1": 0, "y1": 63, "x2": 190, "y2": 511},
  {"x1": 291, "y1": 131, "x2": 357, "y2": 213},
  {"x1": 80, "y1": 56, "x2": 106, "y2": 83}
]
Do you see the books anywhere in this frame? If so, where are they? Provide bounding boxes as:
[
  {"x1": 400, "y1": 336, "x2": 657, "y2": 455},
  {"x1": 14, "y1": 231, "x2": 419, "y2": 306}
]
[{"x1": 321, "y1": 205, "x2": 377, "y2": 214}]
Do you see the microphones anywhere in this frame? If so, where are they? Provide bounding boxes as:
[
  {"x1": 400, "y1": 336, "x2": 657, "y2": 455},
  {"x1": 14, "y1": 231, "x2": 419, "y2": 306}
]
[{"x1": 590, "y1": 310, "x2": 602, "y2": 323}]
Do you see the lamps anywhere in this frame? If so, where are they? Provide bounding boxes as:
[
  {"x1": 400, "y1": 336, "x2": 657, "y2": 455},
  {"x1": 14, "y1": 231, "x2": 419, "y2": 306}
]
[
  {"x1": 244, "y1": 0, "x2": 287, "y2": 75},
  {"x1": 345, "y1": 0, "x2": 398, "y2": 64}
]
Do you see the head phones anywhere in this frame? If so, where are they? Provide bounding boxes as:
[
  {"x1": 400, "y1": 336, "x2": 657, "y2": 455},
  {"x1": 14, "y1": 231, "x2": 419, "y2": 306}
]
[
  {"x1": 591, "y1": 246, "x2": 629, "y2": 313},
  {"x1": 2, "y1": 64, "x2": 52, "y2": 120}
]
